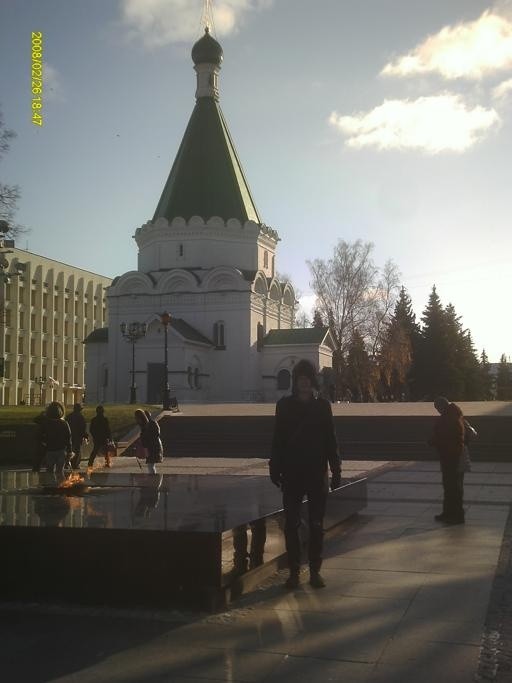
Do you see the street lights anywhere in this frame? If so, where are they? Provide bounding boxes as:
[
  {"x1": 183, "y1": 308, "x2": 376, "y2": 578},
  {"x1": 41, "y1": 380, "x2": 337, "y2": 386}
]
[
  {"x1": 119, "y1": 321, "x2": 149, "y2": 406},
  {"x1": 160, "y1": 310, "x2": 173, "y2": 411},
  {"x1": 35, "y1": 376, "x2": 46, "y2": 405}
]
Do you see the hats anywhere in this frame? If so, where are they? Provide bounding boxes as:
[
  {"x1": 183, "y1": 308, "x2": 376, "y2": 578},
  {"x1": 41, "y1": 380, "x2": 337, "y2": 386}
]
[{"x1": 291, "y1": 359, "x2": 321, "y2": 397}]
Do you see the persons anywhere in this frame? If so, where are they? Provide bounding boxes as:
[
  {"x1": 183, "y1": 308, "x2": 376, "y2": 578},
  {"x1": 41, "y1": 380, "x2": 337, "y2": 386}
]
[
  {"x1": 33, "y1": 402, "x2": 112, "y2": 472},
  {"x1": 136, "y1": 408, "x2": 163, "y2": 474},
  {"x1": 232, "y1": 518, "x2": 266, "y2": 574},
  {"x1": 425, "y1": 397, "x2": 471, "y2": 524},
  {"x1": 329, "y1": 384, "x2": 335, "y2": 403},
  {"x1": 136, "y1": 473, "x2": 163, "y2": 508},
  {"x1": 268, "y1": 358, "x2": 342, "y2": 588}
]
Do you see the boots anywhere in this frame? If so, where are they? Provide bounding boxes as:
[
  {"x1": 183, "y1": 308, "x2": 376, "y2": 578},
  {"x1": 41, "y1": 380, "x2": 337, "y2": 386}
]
[
  {"x1": 434, "y1": 508, "x2": 464, "y2": 524},
  {"x1": 286, "y1": 565, "x2": 300, "y2": 589},
  {"x1": 309, "y1": 557, "x2": 323, "y2": 588}
]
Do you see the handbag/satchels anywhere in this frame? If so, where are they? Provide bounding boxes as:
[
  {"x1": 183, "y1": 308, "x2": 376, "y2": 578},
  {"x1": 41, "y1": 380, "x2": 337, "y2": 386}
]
[
  {"x1": 462, "y1": 417, "x2": 480, "y2": 443},
  {"x1": 438, "y1": 444, "x2": 471, "y2": 473},
  {"x1": 135, "y1": 445, "x2": 149, "y2": 459}
]
